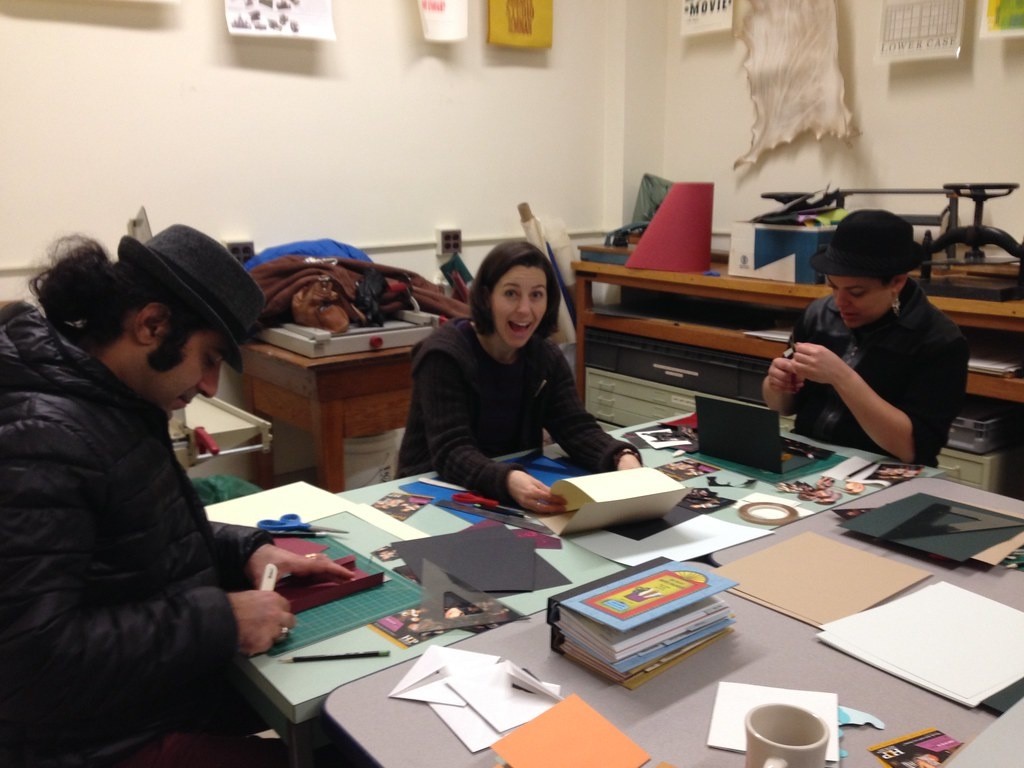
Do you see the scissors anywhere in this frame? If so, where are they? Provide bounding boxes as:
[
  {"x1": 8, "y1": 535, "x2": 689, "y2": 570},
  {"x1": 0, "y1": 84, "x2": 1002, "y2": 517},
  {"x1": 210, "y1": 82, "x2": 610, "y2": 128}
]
[
  {"x1": 257, "y1": 514, "x2": 350, "y2": 534},
  {"x1": 451, "y1": 493, "x2": 525, "y2": 517}
]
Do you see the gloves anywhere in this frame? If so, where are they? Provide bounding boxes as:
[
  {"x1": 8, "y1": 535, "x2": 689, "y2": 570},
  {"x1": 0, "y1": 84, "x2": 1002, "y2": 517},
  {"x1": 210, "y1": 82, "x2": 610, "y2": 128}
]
[{"x1": 356, "y1": 270, "x2": 389, "y2": 327}]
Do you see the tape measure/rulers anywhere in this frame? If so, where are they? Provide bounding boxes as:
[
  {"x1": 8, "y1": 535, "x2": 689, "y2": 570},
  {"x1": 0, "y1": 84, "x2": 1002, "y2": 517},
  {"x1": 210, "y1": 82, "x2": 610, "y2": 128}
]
[{"x1": 436, "y1": 500, "x2": 555, "y2": 534}]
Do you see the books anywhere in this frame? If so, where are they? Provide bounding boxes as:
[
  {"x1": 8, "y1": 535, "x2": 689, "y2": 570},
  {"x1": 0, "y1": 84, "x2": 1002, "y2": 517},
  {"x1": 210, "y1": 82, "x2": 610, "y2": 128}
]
[{"x1": 545, "y1": 553, "x2": 743, "y2": 698}]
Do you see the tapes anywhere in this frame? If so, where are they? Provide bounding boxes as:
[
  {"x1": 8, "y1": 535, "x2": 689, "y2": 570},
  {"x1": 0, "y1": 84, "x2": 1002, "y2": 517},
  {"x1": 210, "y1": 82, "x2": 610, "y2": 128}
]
[{"x1": 736, "y1": 501, "x2": 800, "y2": 526}]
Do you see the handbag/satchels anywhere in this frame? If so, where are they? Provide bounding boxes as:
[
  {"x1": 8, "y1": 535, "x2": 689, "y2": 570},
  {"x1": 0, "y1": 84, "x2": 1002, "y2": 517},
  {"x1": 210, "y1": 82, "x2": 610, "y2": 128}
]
[{"x1": 291, "y1": 282, "x2": 349, "y2": 333}]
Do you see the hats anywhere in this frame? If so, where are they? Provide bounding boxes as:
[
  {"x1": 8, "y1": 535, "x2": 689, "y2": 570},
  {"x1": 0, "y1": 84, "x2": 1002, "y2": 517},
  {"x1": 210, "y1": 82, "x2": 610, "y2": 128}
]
[
  {"x1": 810, "y1": 210, "x2": 926, "y2": 278},
  {"x1": 118, "y1": 223, "x2": 266, "y2": 373}
]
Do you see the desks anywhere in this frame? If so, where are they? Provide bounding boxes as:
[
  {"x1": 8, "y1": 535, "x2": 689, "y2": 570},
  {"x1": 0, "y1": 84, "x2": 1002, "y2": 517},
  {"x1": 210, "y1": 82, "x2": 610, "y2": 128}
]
[
  {"x1": 205, "y1": 414, "x2": 1024, "y2": 768},
  {"x1": 237, "y1": 338, "x2": 414, "y2": 491}
]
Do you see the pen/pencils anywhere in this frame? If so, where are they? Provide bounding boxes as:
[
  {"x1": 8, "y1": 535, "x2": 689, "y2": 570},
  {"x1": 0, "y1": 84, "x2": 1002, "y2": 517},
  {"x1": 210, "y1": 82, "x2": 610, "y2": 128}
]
[{"x1": 277, "y1": 651, "x2": 390, "y2": 664}]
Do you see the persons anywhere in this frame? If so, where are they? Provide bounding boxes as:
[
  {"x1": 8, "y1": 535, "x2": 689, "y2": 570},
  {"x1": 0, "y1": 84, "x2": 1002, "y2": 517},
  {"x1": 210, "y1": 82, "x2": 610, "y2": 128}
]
[
  {"x1": 761, "y1": 206, "x2": 969, "y2": 469},
  {"x1": 392, "y1": 240, "x2": 647, "y2": 516},
  {"x1": 0, "y1": 224, "x2": 360, "y2": 768}
]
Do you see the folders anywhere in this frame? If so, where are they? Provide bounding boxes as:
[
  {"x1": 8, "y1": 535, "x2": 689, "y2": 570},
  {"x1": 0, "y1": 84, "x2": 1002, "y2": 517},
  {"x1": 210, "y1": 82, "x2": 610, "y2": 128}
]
[{"x1": 546, "y1": 556, "x2": 740, "y2": 691}]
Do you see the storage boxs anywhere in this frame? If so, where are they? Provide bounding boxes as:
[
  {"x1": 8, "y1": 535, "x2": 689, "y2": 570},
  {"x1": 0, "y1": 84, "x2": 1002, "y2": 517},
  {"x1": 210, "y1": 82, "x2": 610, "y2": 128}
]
[{"x1": 728, "y1": 221, "x2": 837, "y2": 286}]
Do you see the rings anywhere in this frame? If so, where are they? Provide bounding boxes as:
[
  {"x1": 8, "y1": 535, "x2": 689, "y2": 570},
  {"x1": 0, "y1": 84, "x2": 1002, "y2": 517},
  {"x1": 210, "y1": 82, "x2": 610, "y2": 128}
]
[
  {"x1": 277, "y1": 627, "x2": 289, "y2": 641},
  {"x1": 305, "y1": 552, "x2": 318, "y2": 561},
  {"x1": 534, "y1": 500, "x2": 541, "y2": 511}
]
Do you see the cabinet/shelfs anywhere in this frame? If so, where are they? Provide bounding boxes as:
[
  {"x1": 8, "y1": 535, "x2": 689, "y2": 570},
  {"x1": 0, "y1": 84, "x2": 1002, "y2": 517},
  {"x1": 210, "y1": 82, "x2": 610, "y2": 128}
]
[{"x1": 569, "y1": 258, "x2": 1024, "y2": 496}]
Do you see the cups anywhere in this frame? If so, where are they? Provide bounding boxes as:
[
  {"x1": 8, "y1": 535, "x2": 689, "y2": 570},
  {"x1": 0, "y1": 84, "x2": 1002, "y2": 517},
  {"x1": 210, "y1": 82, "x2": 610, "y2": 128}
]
[{"x1": 745, "y1": 704, "x2": 829, "y2": 768}]
